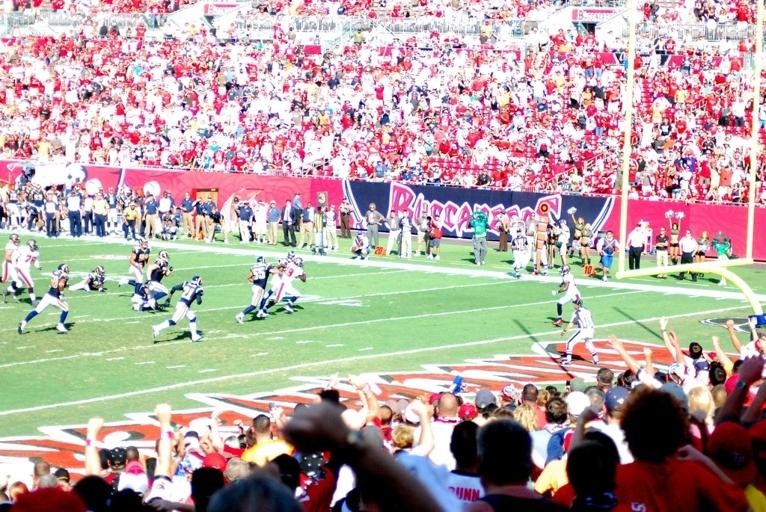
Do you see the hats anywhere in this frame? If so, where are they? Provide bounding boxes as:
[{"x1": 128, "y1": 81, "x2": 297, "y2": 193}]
[
  {"x1": 571, "y1": 299, "x2": 583, "y2": 306},
  {"x1": 604, "y1": 385, "x2": 630, "y2": 412},
  {"x1": 110, "y1": 447, "x2": 127, "y2": 471},
  {"x1": 660, "y1": 384, "x2": 688, "y2": 412},
  {"x1": 475, "y1": 389, "x2": 499, "y2": 409}
]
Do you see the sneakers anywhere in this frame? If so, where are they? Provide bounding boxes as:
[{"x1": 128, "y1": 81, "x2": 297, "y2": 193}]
[
  {"x1": 118, "y1": 277, "x2": 160, "y2": 312},
  {"x1": 56, "y1": 324, "x2": 68, "y2": 333},
  {"x1": 18, "y1": 320, "x2": 26, "y2": 333},
  {"x1": 553, "y1": 320, "x2": 564, "y2": 326},
  {"x1": 0, "y1": 218, "x2": 728, "y2": 290},
  {"x1": 1, "y1": 288, "x2": 39, "y2": 306},
  {"x1": 234, "y1": 303, "x2": 297, "y2": 325},
  {"x1": 192, "y1": 335, "x2": 204, "y2": 343},
  {"x1": 152, "y1": 324, "x2": 159, "y2": 339},
  {"x1": 561, "y1": 358, "x2": 599, "y2": 366}
]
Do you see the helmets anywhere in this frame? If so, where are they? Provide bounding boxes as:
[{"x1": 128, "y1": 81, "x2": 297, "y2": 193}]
[
  {"x1": 257, "y1": 256, "x2": 265, "y2": 261},
  {"x1": 9, "y1": 233, "x2": 22, "y2": 246},
  {"x1": 26, "y1": 239, "x2": 39, "y2": 252},
  {"x1": 191, "y1": 276, "x2": 203, "y2": 287},
  {"x1": 139, "y1": 238, "x2": 148, "y2": 249},
  {"x1": 287, "y1": 251, "x2": 303, "y2": 266},
  {"x1": 158, "y1": 249, "x2": 170, "y2": 262},
  {"x1": 558, "y1": 264, "x2": 570, "y2": 274},
  {"x1": 96, "y1": 265, "x2": 105, "y2": 276},
  {"x1": 58, "y1": 263, "x2": 70, "y2": 273}
]
